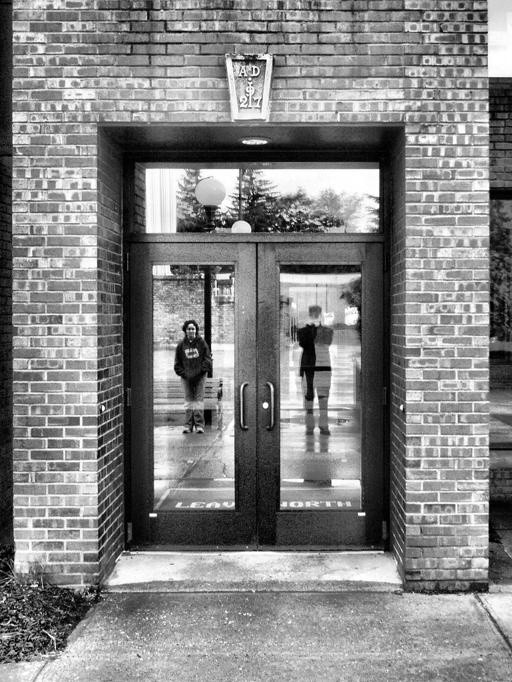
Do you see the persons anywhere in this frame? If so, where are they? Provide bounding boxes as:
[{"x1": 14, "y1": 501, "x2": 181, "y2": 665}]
[
  {"x1": 172, "y1": 318, "x2": 214, "y2": 436},
  {"x1": 296, "y1": 305, "x2": 336, "y2": 436}
]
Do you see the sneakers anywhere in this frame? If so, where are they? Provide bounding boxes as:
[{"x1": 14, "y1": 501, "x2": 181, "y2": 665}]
[
  {"x1": 306, "y1": 429, "x2": 330, "y2": 436},
  {"x1": 182, "y1": 426, "x2": 192, "y2": 433},
  {"x1": 196, "y1": 427, "x2": 204, "y2": 433}
]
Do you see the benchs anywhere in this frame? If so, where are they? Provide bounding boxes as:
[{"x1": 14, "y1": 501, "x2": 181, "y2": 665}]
[{"x1": 150, "y1": 379, "x2": 221, "y2": 429}]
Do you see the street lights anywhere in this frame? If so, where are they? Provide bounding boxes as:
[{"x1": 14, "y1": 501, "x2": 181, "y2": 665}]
[{"x1": 194, "y1": 176, "x2": 227, "y2": 426}]
